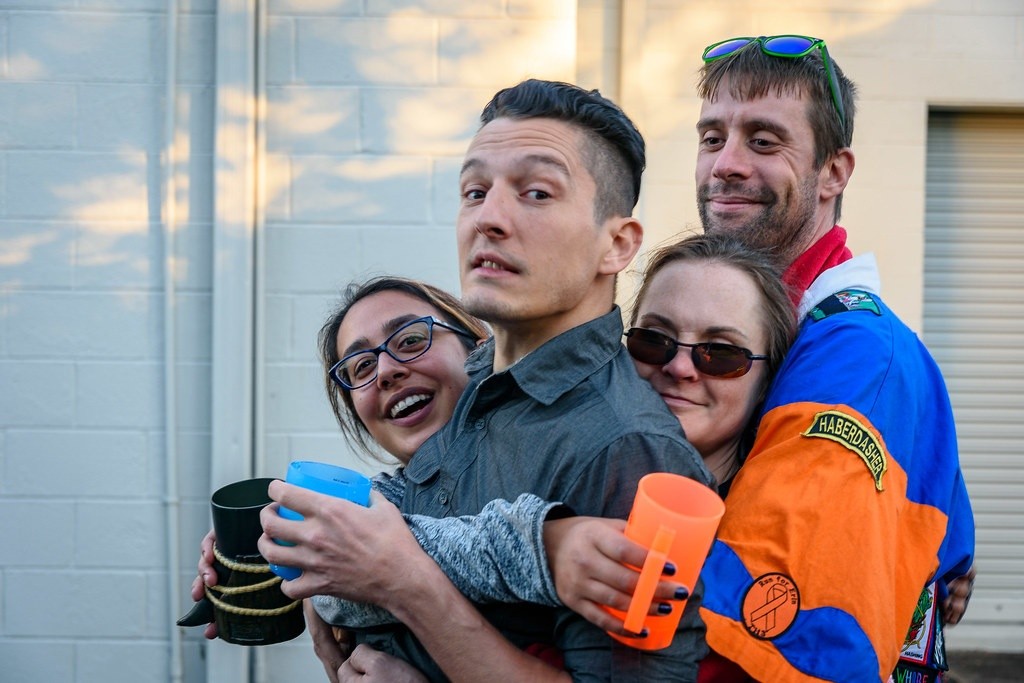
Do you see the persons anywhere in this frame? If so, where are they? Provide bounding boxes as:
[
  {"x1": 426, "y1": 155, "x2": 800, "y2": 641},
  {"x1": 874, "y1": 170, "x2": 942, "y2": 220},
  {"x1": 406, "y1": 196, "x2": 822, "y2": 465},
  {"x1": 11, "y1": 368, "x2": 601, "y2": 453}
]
[{"x1": 192, "y1": 35, "x2": 974, "y2": 683}]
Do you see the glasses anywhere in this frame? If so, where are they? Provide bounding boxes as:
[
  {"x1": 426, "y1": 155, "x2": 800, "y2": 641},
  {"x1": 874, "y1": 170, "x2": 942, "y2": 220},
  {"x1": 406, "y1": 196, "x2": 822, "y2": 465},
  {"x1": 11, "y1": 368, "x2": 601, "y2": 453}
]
[
  {"x1": 623, "y1": 327, "x2": 770, "y2": 378},
  {"x1": 702, "y1": 35, "x2": 844, "y2": 129},
  {"x1": 327, "y1": 315, "x2": 476, "y2": 391}
]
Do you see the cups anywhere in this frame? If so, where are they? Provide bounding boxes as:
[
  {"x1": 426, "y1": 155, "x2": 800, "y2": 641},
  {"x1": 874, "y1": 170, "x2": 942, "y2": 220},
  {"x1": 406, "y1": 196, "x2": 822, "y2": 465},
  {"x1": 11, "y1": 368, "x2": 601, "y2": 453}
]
[
  {"x1": 600, "y1": 473, "x2": 725, "y2": 648},
  {"x1": 268, "y1": 460, "x2": 372, "y2": 580},
  {"x1": 204, "y1": 476, "x2": 307, "y2": 646}
]
